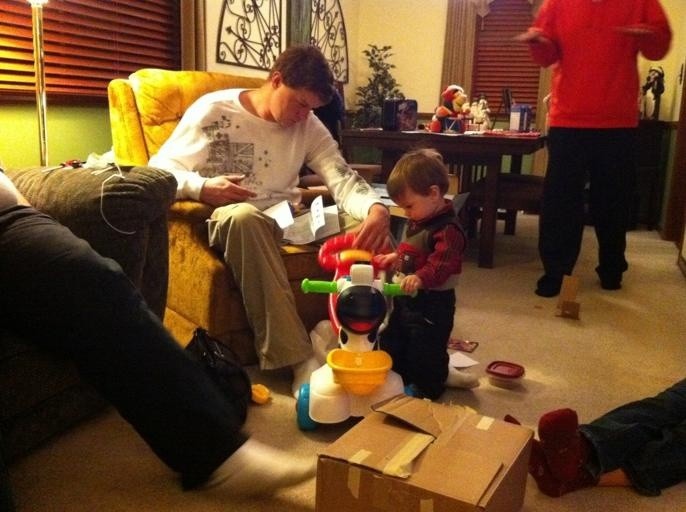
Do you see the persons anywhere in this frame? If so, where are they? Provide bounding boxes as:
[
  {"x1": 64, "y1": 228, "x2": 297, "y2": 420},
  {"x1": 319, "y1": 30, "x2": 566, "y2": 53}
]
[
  {"x1": 374, "y1": 143, "x2": 467, "y2": 405},
  {"x1": 502, "y1": 370, "x2": 686, "y2": 497},
  {"x1": 147, "y1": 41, "x2": 393, "y2": 406},
  {"x1": 0, "y1": 166, "x2": 317, "y2": 500},
  {"x1": 513, "y1": 1, "x2": 677, "y2": 299}
]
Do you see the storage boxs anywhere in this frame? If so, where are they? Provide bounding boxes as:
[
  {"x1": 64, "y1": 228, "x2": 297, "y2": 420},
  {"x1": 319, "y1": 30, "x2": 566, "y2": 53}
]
[
  {"x1": 357, "y1": 167, "x2": 470, "y2": 243},
  {"x1": 316, "y1": 394, "x2": 534, "y2": 512}
]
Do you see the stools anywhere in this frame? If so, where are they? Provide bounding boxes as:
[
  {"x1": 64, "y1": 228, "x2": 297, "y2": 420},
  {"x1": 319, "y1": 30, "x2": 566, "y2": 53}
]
[{"x1": 469, "y1": 172, "x2": 549, "y2": 259}]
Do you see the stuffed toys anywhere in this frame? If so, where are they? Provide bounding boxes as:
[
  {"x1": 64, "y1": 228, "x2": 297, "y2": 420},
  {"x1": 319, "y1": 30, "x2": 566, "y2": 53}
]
[{"x1": 427, "y1": 81, "x2": 473, "y2": 137}]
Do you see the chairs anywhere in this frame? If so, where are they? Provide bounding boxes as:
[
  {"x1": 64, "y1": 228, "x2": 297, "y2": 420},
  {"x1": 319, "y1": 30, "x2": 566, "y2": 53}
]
[
  {"x1": 0, "y1": 165, "x2": 179, "y2": 469},
  {"x1": 106, "y1": 67, "x2": 376, "y2": 365}
]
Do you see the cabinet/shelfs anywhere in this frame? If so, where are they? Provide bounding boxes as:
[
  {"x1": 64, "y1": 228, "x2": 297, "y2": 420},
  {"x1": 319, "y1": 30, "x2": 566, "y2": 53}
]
[{"x1": 583, "y1": 116, "x2": 678, "y2": 232}]
